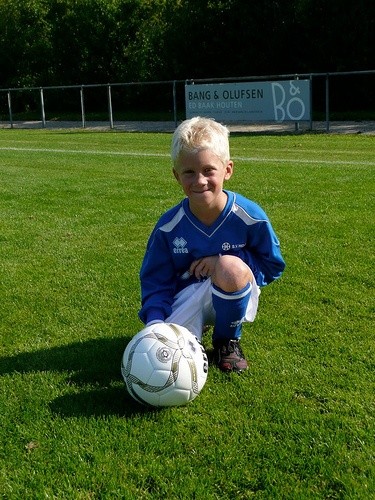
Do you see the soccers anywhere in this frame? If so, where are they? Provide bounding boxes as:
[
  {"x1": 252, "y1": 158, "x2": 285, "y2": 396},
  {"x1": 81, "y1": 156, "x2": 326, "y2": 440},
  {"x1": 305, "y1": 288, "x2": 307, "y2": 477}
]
[{"x1": 121, "y1": 323, "x2": 209, "y2": 407}]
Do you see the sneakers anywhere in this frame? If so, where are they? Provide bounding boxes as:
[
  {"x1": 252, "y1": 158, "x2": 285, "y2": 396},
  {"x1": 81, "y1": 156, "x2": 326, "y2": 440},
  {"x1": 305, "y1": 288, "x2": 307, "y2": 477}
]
[{"x1": 212, "y1": 331, "x2": 249, "y2": 372}]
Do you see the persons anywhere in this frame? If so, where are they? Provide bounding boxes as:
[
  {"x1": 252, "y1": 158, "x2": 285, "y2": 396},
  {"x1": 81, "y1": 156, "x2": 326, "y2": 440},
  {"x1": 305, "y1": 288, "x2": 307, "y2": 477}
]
[{"x1": 137, "y1": 115, "x2": 286, "y2": 374}]
